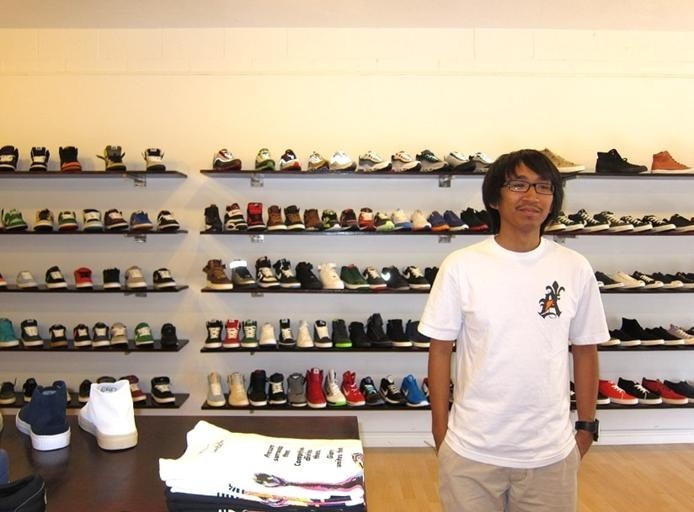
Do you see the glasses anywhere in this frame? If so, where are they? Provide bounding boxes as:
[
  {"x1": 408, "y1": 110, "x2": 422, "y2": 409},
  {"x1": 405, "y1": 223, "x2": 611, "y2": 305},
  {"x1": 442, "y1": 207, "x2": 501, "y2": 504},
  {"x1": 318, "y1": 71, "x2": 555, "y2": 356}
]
[{"x1": 500, "y1": 181, "x2": 555, "y2": 195}]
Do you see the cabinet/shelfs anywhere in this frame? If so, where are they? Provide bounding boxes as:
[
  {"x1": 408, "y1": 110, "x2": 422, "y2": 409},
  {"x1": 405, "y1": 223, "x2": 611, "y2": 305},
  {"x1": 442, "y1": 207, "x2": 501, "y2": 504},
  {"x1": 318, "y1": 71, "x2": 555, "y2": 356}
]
[
  {"x1": 0, "y1": 170, "x2": 191, "y2": 409},
  {"x1": 200, "y1": 169, "x2": 693, "y2": 414}
]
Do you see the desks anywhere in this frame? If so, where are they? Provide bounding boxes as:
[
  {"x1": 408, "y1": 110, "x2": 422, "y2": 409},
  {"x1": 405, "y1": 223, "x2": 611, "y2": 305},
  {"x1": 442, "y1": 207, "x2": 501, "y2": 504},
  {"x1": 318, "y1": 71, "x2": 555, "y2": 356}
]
[{"x1": 0, "y1": 414, "x2": 367, "y2": 512}]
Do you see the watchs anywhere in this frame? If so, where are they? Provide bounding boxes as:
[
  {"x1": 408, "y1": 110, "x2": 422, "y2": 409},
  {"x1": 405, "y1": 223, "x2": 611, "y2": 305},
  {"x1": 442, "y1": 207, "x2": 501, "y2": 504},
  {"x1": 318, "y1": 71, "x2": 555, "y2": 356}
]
[{"x1": 575, "y1": 419, "x2": 599, "y2": 442}]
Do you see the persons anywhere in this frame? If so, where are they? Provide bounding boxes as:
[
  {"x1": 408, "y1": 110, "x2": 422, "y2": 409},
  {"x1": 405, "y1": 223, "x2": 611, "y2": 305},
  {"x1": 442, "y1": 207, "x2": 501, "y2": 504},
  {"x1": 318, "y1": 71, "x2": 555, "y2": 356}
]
[{"x1": 418, "y1": 148, "x2": 611, "y2": 512}]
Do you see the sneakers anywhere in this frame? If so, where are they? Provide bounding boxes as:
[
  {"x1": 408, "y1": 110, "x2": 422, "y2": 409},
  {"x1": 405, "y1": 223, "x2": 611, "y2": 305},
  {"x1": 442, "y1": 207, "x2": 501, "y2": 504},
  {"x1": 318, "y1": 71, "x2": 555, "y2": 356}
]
[
  {"x1": 596, "y1": 271, "x2": 694, "y2": 288},
  {"x1": 541, "y1": 149, "x2": 694, "y2": 175},
  {"x1": 570, "y1": 378, "x2": 694, "y2": 405},
  {"x1": 203, "y1": 257, "x2": 440, "y2": 291},
  {"x1": 1, "y1": 319, "x2": 180, "y2": 347},
  {"x1": 96, "y1": 145, "x2": 126, "y2": 171},
  {"x1": 545, "y1": 208, "x2": 693, "y2": 233},
  {"x1": 204, "y1": 202, "x2": 491, "y2": 232},
  {"x1": 16, "y1": 381, "x2": 71, "y2": 451},
  {"x1": 59, "y1": 146, "x2": 81, "y2": 171},
  {"x1": 0, "y1": 376, "x2": 176, "y2": 405},
  {"x1": 0, "y1": 146, "x2": 18, "y2": 172},
  {"x1": 203, "y1": 314, "x2": 431, "y2": 348},
  {"x1": 0, "y1": 472, "x2": 47, "y2": 512},
  {"x1": 213, "y1": 148, "x2": 491, "y2": 174},
  {"x1": 601, "y1": 318, "x2": 694, "y2": 346},
  {"x1": 0, "y1": 266, "x2": 176, "y2": 288},
  {"x1": 208, "y1": 370, "x2": 432, "y2": 407},
  {"x1": 29, "y1": 146, "x2": 49, "y2": 171},
  {"x1": 1, "y1": 208, "x2": 180, "y2": 231},
  {"x1": 78, "y1": 380, "x2": 139, "y2": 450},
  {"x1": 141, "y1": 148, "x2": 166, "y2": 170}
]
